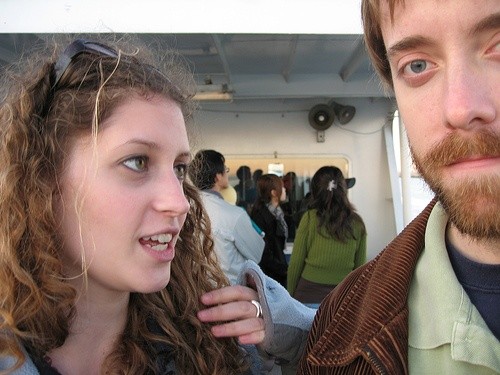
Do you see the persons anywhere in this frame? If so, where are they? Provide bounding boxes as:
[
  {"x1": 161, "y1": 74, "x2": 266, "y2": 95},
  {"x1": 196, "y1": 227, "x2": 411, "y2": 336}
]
[
  {"x1": 188, "y1": 149, "x2": 368, "y2": 305},
  {"x1": 0, "y1": 33, "x2": 317, "y2": 375},
  {"x1": 299, "y1": 0, "x2": 500, "y2": 375}
]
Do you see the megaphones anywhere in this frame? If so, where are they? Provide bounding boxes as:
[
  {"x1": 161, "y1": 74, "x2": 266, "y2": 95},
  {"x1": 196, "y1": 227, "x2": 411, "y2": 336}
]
[
  {"x1": 330, "y1": 102, "x2": 355, "y2": 124},
  {"x1": 308, "y1": 105, "x2": 334, "y2": 130}
]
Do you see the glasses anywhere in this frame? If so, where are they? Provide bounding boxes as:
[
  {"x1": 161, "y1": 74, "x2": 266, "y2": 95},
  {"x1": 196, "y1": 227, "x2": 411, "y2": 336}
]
[
  {"x1": 225, "y1": 168, "x2": 230, "y2": 173},
  {"x1": 41, "y1": 41, "x2": 118, "y2": 90}
]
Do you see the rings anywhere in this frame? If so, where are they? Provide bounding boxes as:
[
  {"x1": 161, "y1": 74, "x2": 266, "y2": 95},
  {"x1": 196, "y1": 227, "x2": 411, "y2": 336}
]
[{"x1": 250, "y1": 299, "x2": 264, "y2": 319}]
